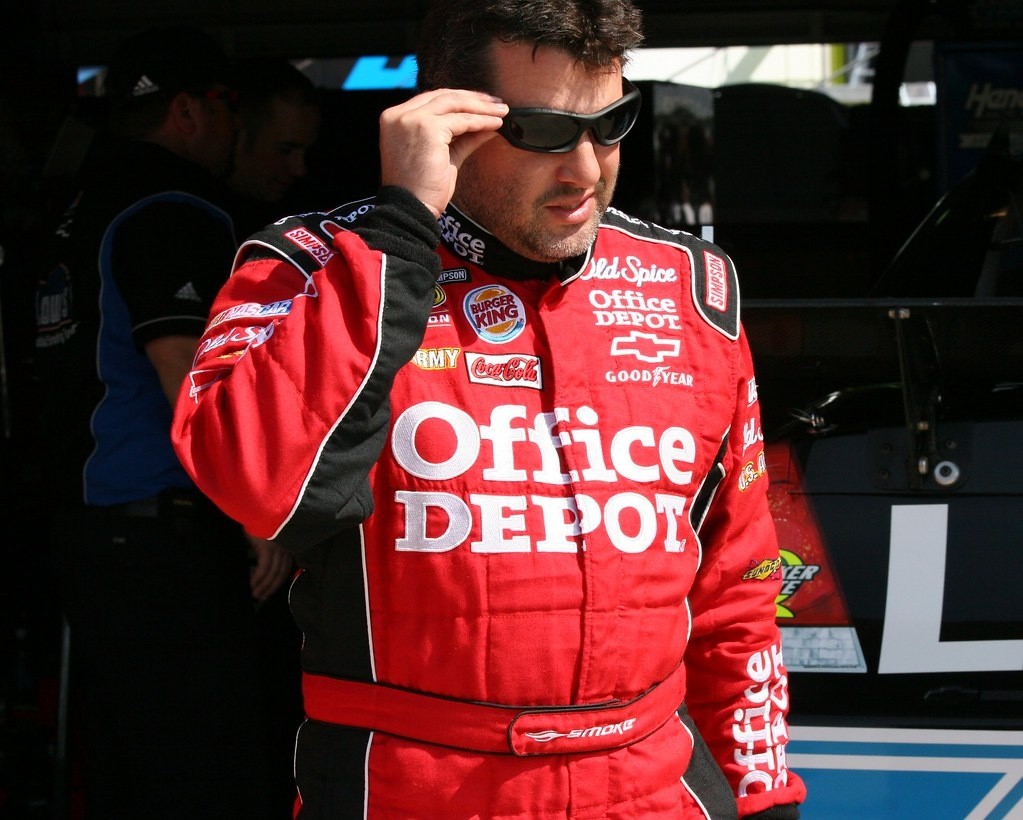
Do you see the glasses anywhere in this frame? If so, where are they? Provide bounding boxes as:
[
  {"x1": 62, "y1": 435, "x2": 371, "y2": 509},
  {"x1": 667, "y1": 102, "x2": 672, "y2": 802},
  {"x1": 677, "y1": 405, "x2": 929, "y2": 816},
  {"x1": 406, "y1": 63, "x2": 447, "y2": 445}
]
[
  {"x1": 185, "y1": 89, "x2": 237, "y2": 110},
  {"x1": 493, "y1": 75, "x2": 643, "y2": 154}
]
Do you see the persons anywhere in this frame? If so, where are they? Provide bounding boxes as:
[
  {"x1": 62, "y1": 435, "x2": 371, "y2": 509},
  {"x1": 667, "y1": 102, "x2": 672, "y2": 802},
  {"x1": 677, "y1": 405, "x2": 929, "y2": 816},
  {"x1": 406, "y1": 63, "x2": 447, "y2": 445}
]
[
  {"x1": 169, "y1": 0, "x2": 806, "y2": 820},
  {"x1": 53, "y1": 23, "x2": 323, "y2": 820}
]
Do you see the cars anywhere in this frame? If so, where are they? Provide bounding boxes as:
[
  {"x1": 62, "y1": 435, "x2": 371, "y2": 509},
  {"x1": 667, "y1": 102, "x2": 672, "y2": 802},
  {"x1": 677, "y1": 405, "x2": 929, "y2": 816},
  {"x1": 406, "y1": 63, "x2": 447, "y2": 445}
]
[{"x1": 731, "y1": 295, "x2": 1023, "y2": 627}]
